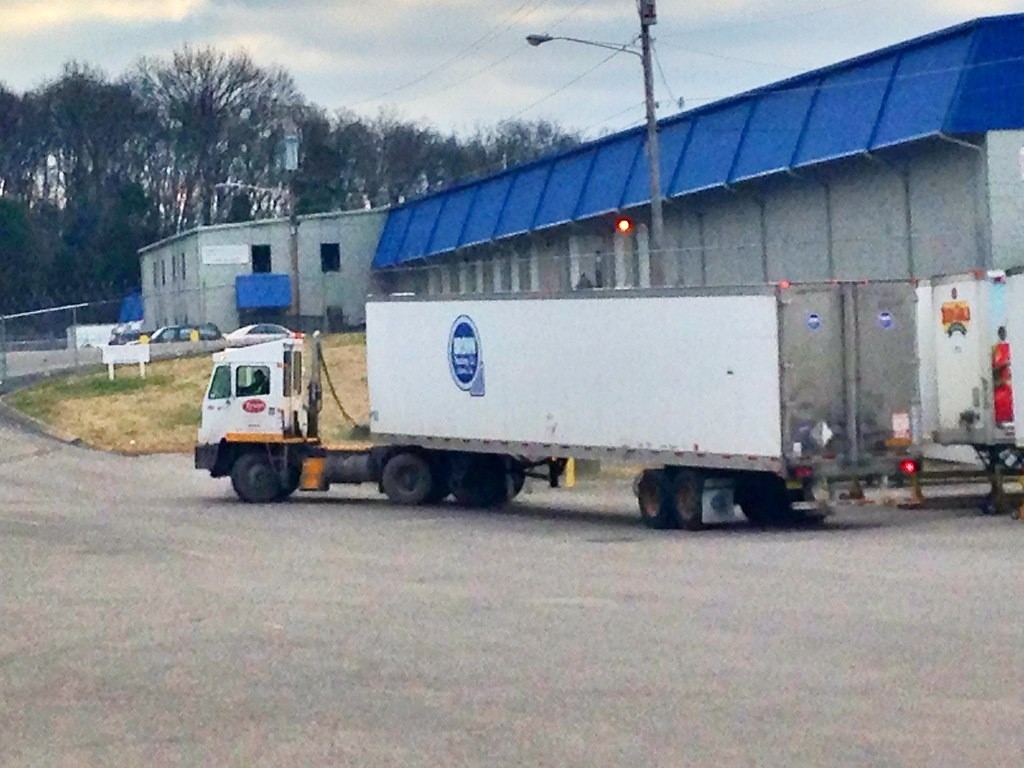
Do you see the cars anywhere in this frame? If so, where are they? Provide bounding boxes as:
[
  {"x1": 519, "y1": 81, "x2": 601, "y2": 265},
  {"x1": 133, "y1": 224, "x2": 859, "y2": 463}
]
[{"x1": 216, "y1": 323, "x2": 303, "y2": 341}]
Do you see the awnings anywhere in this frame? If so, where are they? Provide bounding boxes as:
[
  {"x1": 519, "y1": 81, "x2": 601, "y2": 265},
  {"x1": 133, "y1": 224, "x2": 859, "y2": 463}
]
[
  {"x1": 118, "y1": 295, "x2": 142, "y2": 323},
  {"x1": 371, "y1": 14, "x2": 1024, "y2": 269},
  {"x1": 236, "y1": 275, "x2": 291, "y2": 310}
]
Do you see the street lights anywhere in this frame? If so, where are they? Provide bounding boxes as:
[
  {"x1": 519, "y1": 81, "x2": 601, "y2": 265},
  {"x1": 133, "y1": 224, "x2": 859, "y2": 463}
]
[
  {"x1": 216, "y1": 182, "x2": 299, "y2": 334},
  {"x1": 527, "y1": 35, "x2": 665, "y2": 290}
]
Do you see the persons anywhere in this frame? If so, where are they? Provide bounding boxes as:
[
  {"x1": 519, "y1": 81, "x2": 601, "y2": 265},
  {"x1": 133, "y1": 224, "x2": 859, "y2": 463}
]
[
  {"x1": 595, "y1": 250, "x2": 603, "y2": 288},
  {"x1": 574, "y1": 272, "x2": 593, "y2": 290},
  {"x1": 239, "y1": 369, "x2": 269, "y2": 396}
]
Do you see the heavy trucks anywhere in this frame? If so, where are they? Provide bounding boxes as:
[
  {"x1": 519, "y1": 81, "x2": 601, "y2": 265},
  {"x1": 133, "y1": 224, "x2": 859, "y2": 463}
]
[{"x1": 193, "y1": 276, "x2": 919, "y2": 531}]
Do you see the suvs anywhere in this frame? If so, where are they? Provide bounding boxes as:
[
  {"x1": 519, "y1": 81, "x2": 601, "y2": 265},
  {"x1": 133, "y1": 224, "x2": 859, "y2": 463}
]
[{"x1": 127, "y1": 321, "x2": 220, "y2": 343}]
